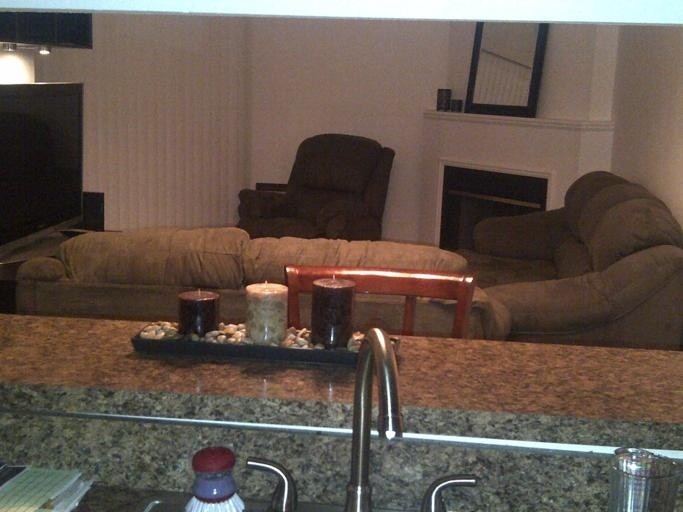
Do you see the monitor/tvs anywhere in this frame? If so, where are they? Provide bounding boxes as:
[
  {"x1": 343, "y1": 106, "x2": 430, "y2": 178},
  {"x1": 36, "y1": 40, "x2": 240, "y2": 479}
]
[{"x1": 0, "y1": 80, "x2": 84, "y2": 264}]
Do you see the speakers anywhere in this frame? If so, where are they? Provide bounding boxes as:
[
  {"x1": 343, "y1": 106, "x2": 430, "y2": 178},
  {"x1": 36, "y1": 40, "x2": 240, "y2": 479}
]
[{"x1": 79, "y1": 190, "x2": 104, "y2": 229}]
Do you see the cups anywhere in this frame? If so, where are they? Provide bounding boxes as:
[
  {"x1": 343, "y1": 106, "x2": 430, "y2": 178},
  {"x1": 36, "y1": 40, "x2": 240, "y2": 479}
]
[
  {"x1": 608, "y1": 451, "x2": 681, "y2": 511},
  {"x1": 436, "y1": 88, "x2": 451, "y2": 110},
  {"x1": 450, "y1": 99, "x2": 463, "y2": 112}
]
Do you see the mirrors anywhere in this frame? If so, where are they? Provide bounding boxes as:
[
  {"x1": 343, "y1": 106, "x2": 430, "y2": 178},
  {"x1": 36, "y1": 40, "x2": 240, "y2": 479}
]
[{"x1": 461, "y1": 23, "x2": 548, "y2": 120}]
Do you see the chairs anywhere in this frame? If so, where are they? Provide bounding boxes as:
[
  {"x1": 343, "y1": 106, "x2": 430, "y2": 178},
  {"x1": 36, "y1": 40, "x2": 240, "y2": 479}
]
[{"x1": 236, "y1": 133, "x2": 396, "y2": 242}]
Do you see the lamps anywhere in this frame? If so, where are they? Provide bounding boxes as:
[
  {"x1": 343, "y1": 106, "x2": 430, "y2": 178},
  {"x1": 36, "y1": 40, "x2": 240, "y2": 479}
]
[{"x1": 2, "y1": 43, "x2": 51, "y2": 55}]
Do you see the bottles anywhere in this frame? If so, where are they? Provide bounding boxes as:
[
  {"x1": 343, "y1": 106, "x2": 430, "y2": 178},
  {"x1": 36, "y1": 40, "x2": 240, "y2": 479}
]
[{"x1": 184, "y1": 447, "x2": 245, "y2": 512}]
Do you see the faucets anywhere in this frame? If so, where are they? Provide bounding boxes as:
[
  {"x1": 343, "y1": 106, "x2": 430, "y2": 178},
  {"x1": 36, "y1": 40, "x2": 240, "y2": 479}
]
[{"x1": 344, "y1": 326, "x2": 402, "y2": 512}]
